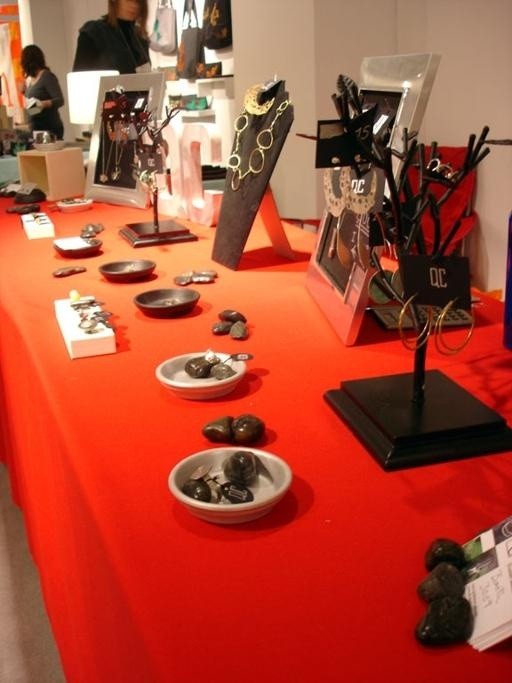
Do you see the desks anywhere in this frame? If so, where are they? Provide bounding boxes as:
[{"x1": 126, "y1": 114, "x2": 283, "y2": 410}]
[{"x1": 2, "y1": 174, "x2": 512, "y2": 681}]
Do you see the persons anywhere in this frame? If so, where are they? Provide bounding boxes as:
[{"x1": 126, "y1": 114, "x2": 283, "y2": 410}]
[
  {"x1": 69, "y1": 0, "x2": 149, "y2": 74},
  {"x1": 19, "y1": 43, "x2": 66, "y2": 140}
]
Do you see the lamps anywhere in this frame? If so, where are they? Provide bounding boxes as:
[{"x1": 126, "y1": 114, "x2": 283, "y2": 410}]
[{"x1": 66, "y1": 68, "x2": 118, "y2": 130}]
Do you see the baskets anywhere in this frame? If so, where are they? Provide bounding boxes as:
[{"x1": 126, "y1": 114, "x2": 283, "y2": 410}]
[{"x1": 193, "y1": 61, "x2": 222, "y2": 78}]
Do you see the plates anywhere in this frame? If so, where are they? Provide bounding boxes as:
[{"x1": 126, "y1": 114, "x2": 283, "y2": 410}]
[
  {"x1": 99, "y1": 259, "x2": 156, "y2": 282},
  {"x1": 53, "y1": 235, "x2": 104, "y2": 258},
  {"x1": 168, "y1": 445, "x2": 294, "y2": 525},
  {"x1": 56, "y1": 196, "x2": 94, "y2": 213},
  {"x1": 155, "y1": 350, "x2": 247, "y2": 401},
  {"x1": 132, "y1": 286, "x2": 200, "y2": 317},
  {"x1": 34, "y1": 140, "x2": 67, "y2": 150}
]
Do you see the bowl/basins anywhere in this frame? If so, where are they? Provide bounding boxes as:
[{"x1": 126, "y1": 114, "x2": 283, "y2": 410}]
[{"x1": 36, "y1": 132, "x2": 57, "y2": 144}]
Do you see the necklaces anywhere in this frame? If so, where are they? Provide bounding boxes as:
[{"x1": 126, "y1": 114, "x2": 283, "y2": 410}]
[
  {"x1": 99, "y1": 121, "x2": 116, "y2": 183},
  {"x1": 112, "y1": 140, "x2": 125, "y2": 181},
  {"x1": 228, "y1": 93, "x2": 291, "y2": 191}
]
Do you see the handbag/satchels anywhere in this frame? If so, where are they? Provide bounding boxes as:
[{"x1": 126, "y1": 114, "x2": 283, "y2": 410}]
[
  {"x1": 149, "y1": 0, "x2": 176, "y2": 54},
  {"x1": 175, "y1": 0, "x2": 206, "y2": 78},
  {"x1": 200, "y1": 0, "x2": 232, "y2": 49}
]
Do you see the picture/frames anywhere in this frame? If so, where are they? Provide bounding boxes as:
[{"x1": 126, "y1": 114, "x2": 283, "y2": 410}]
[
  {"x1": 305, "y1": 50, "x2": 442, "y2": 347},
  {"x1": 82, "y1": 70, "x2": 166, "y2": 210}
]
